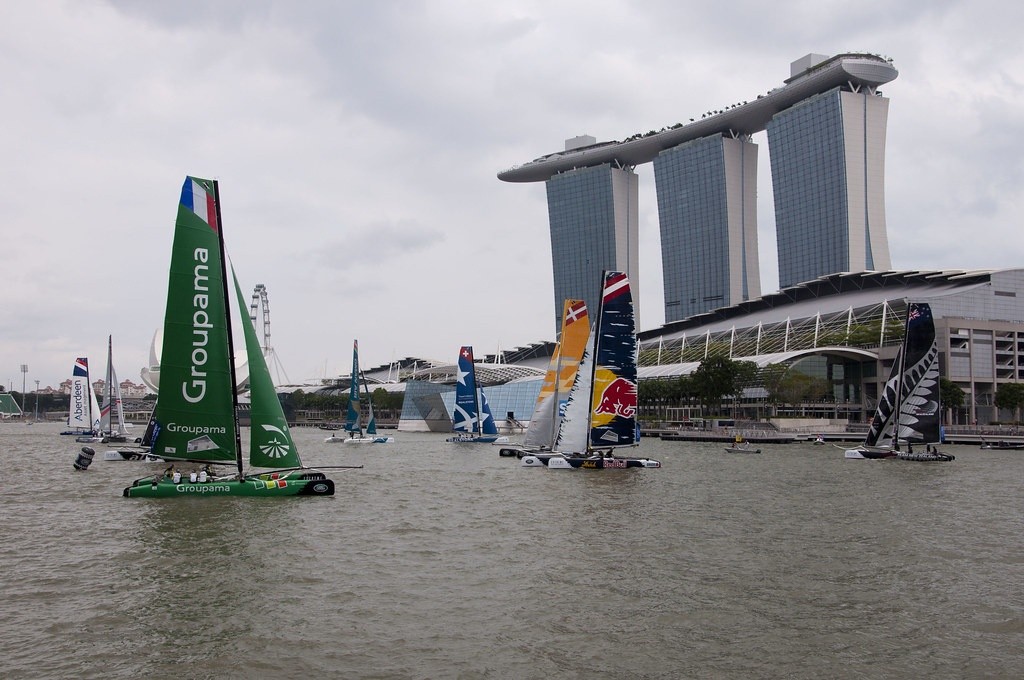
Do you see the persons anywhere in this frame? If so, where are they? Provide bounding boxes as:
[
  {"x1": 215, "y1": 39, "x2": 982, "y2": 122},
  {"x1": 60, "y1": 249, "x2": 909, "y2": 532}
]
[
  {"x1": 350, "y1": 430, "x2": 355, "y2": 439},
  {"x1": 972, "y1": 417, "x2": 1019, "y2": 435},
  {"x1": 598, "y1": 447, "x2": 614, "y2": 459},
  {"x1": 93, "y1": 429, "x2": 103, "y2": 439},
  {"x1": 459, "y1": 432, "x2": 474, "y2": 438},
  {"x1": 722, "y1": 425, "x2": 749, "y2": 450},
  {"x1": 907, "y1": 444, "x2": 938, "y2": 455},
  {"x1": 164, "y1": 464, "x2": 212, "y2": 484}
]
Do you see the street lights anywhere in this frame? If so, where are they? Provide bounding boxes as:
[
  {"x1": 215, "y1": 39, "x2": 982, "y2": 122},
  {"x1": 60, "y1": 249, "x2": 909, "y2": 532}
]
[
  {"x1": 20, "y1": 364, "x2": 29, "y2": 421},
  {"x1": 33, "y1": 379, "x2": 41, "y2": 423}
]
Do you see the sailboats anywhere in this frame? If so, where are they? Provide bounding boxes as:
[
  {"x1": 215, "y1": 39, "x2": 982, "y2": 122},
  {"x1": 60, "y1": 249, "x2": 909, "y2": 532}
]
[
  {"x1": 444, "y1": 270, "x2": 662, "y2": 472},
  {"x1": 845, "y1": 302, "x2": 956, "y2": 462},
  {"x1": 324, "y1": 338, "x2": 395, "y2": 445},
  {"x1": 57, "y1": 174, "x2": 337, "y2": 501}
]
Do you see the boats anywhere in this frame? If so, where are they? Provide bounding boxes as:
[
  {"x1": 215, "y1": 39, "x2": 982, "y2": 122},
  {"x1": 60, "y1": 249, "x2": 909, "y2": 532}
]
[
  {"x1": 813, "y1": 438, "x2": 824, "y2": 445},
  {"x1": 724, "y1": 444, "x2": 762, "y2": 454},
  {"x1": 980, "y1": 444, "x2": 1024, "y2": 449}
]
